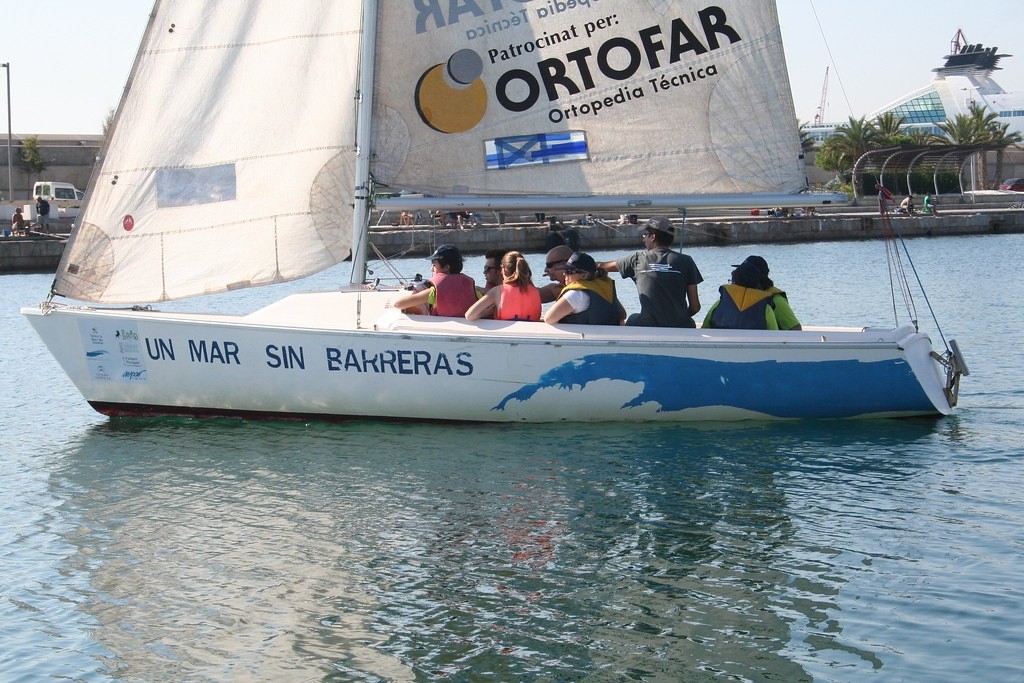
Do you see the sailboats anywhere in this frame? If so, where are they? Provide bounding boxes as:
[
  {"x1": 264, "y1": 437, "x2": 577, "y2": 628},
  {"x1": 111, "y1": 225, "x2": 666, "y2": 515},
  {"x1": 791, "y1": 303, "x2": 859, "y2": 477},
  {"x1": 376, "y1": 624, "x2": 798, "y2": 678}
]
[{"x1": 19, "y1": 0, "x2": 970, "y2": 421}]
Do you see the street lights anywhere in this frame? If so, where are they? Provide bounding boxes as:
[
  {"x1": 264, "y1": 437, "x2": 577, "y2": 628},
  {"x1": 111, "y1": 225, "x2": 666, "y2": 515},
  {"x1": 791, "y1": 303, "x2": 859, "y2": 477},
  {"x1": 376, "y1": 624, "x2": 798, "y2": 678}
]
[{"x1": 0, "y1": 60, "x2": 15, "y2": 201}]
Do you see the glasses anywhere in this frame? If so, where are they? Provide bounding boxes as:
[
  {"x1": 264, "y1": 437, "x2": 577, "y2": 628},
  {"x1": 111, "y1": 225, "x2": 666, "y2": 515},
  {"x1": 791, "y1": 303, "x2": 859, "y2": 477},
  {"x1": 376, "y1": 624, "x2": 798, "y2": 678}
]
[
  {"x1": 545, "y1": 259, "x2": 567, "y2": 270},
  {"x1": 565, "y1": 270, "x2": 586, "y2": 275},
  {"x1": 641, "y1": 230, "x2": 659, "y2": 238},
  {"x1": 482, "y1": 266, "x2": 501, "y2": 274}
]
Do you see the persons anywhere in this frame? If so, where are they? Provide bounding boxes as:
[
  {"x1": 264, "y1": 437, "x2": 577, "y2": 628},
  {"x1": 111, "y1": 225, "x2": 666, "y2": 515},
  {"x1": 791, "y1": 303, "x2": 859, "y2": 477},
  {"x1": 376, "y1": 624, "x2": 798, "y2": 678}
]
[
  {"x1": 801, "y1": 207, "x2": 815, "y2": 215},
  {"x1": 536, "y1": 246, "x2": 625, "y2": 324},
  {"x1": 11, "y1": 207, "x2": 26, "y2": 231},
  {"x1": 465, "y1": 250, "x2": 542, "y2": 321},
  {"x1": 701, "y1": 256, "x2": 802, "y2": 329},
  {"x1": 924, "y1": 192, "x2": 937, "y2": 217},
  {"x1": 900, "y1": 195, "x2": 914, "y2": 216},
  {"x1": 544, "y1": 252, "x2": 627, "y2": 325},
  {"x1": 35, "y1": 197, "x2": 50, "y2": 234},
  {"x1": 473, "y1": 249, "x2": 507, "y2": 295},
  {"x1": 593, "y1": 216, "x2": 704, "y2": 328},
  {"x1": 774, "y1": 206, "x2": 792, "y2": 218},
  {"x1": 391, "y1": 242, "x2": 481, "y2": 318}
]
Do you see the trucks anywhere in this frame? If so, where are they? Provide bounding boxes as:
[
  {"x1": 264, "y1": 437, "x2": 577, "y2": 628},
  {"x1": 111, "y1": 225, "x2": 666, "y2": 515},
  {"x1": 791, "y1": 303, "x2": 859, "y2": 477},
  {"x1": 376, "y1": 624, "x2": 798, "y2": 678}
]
[{"x1": 33, "y1": 182, "x2": 85, "y2": 200}]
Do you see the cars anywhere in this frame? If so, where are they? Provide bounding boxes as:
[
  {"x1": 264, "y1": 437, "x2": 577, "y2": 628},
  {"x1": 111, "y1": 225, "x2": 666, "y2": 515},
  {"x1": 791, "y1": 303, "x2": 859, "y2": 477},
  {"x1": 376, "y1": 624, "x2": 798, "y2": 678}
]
[{"x1": 997, "y1": 178, "x2": 1024, "y2": 191}]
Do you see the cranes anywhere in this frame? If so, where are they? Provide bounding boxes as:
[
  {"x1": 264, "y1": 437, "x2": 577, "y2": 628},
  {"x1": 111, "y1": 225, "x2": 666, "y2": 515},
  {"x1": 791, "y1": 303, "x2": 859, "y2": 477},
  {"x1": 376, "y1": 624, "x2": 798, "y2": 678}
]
[{"x1": 814, "y1": 65, "x2": 829, "y2": 123}]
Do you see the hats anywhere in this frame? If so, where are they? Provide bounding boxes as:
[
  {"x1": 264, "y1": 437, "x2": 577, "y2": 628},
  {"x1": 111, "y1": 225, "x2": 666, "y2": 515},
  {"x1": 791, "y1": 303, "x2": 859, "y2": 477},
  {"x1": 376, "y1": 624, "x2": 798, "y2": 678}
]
[
  {"x1": 425, "y1": 242, "x2": 463, "y2": 261},
  {"x1": 555, "y1": 252, "x2": 596, "y2": 275},
  {"x1": 732, "y1": 255, "x2": 769, "y2": 273},
  {"x1": 637, "y1": 215, "x2": 676, "y2": 237}
]
